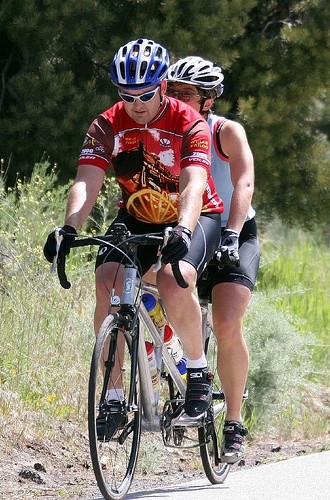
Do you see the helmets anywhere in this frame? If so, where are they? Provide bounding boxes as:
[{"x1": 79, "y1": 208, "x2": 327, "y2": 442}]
[
  {"x1": 110, "y1": 39, "x2": 169, "y2": 91},
  {"x1": 166, "y1": 56, "x2": 224, "y2": 98}
]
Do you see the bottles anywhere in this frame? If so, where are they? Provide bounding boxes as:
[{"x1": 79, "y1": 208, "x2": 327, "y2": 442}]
[
  {"x1": 139, "y1": 294, "x2": 167, "y2": 334},
  {"x1": 163, "y1": 325, "x2": 183, "y2": 366},
  {"x1": 176, "y1": 358, "x2": 189, "y2": 387},
  {"x1": 144, "y1": 340, "x2": 160, "y2": 394}
]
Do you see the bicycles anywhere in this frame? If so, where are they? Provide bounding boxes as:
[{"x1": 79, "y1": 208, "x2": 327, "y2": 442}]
[{"x1": 51, "y1": 224, "x2": 249, "y2": 500}]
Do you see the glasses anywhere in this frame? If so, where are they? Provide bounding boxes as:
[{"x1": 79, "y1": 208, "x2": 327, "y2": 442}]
[
  {"x1": 166, "y1": 89, "x2": 208, "y2": 102},
  {"x1": 117, "y1": 85, "x2": 160, "y2": 104}
]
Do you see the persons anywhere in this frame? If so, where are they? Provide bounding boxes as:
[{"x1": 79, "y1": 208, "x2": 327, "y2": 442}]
[
  {"x1": 165, "y1": 55, "x2": 260, "y2": 464},
  {"x1": 44, "y1": 38, "x2": 224, "y2": 444}
]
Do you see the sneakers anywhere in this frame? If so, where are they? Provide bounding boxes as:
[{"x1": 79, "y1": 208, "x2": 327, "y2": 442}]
[
  {"x1": 96, "y1": 399, "x2": 128, "y2": 442},
  {"x1": 223, "y1": 421, "x2": 246, "y2": 463},
  {"x1": 185, "y1": 366, "x2": 215, "y2": 420}
]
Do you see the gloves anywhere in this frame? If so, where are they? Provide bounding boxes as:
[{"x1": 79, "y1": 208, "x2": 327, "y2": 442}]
[
  {"x1": 43, "y1": 225, "x2": 77, "y2": 263},
  {"x1": 159, "y1": 226, "x2": 192, "y2": 265},
  {"x1": 215, "y1": 229, "x2": 240, "y2": 269}
]
